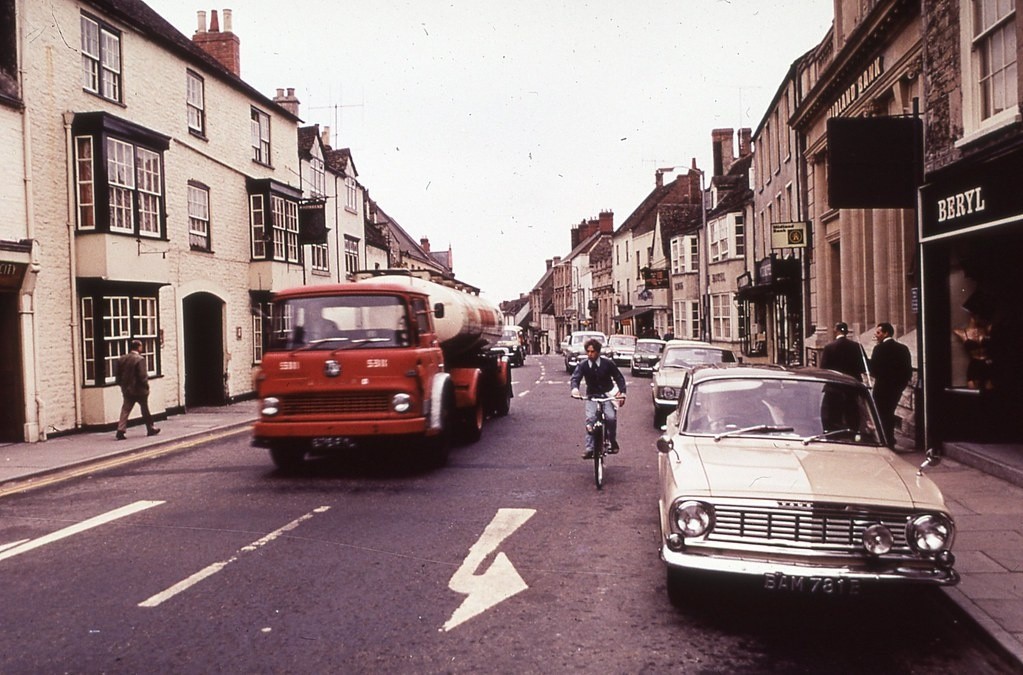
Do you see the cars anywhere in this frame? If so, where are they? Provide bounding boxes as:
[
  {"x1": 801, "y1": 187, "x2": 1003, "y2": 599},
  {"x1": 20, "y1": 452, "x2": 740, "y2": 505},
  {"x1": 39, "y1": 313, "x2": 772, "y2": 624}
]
[
  {"x1": 609, "y1": 334, "x2": 638, "y2": 367},
  {"x1": 491, "y1": 322, "x2": 531, "y2": 369},
  {"x1": 649, "y1": 345, "x2": 745, "y2": 433},
  {"x1": 559, "y1": 331, "x2": 610, "y2": 375},
  {"x1": 631, "y1": 338, "x2": 668, "y2": 377},
  {"x1": 656, "y1": 362, "x2": 963, "y2": 608}
]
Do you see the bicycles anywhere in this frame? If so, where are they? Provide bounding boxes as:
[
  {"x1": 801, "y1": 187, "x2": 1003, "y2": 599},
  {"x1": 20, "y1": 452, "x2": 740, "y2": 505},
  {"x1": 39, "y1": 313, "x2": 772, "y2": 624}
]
[{"x1": 570, "y1": 393, "x2": 628, "y2": 490}]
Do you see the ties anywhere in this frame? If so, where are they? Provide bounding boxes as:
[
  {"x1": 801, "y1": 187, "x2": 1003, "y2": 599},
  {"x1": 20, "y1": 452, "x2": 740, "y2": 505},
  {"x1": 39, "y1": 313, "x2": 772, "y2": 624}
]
[{"x1": 591, "y1": 361, "x2": 597, "y2": 372}]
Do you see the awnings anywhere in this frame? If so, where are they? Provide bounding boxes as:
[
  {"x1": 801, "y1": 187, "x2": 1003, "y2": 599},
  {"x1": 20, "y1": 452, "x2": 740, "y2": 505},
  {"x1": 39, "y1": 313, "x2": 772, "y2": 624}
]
[
  {"x1": 611, "y1": 308, "x2": 652, "y2": 319},
  {"x1": 734, "y1": 280, "x2": 801, "y2": 303}
]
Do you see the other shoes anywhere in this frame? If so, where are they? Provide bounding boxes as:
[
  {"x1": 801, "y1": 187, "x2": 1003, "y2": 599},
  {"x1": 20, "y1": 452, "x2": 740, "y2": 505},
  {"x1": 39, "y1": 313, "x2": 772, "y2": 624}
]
[
  {"x1": 116, "y1": 431, "x2": 126, "y2": 439},
  {"x1": 581, "y1": 450, "x2": 592, "y2": 458},
  {"x1": 147, "y1": 428, "x2": 159, "y2": 436},
  {"x1": 611, "y1": 440, "x2": 618, "y2": 452}
]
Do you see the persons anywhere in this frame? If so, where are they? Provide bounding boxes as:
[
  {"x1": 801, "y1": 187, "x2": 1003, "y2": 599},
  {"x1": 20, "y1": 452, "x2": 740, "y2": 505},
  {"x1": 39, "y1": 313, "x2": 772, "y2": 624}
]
[
  {"x1": 116, "y1": 341, "x2": 160, "y2": 439},
  {"x1": 570, "y1": 339, "x2": 626, "y2": 458},
  {"x1": 639, "y1": 326, "x2": 661, "y2": 339},
  {"x1": 302, "y1": 301, "x2": 338, "y2": 348},
  {"x1": 616, "y1": 329, "x2": 622, "y2": 334},
  {"x1": 821, "y1": 323, "x2": 866, "y2": 383},
  {"x1": 868, "y1": 323, "x2": 912, "y2": 448},
  {"x1": 663, "y1": 329, "x2": 673, "y2": 341}
]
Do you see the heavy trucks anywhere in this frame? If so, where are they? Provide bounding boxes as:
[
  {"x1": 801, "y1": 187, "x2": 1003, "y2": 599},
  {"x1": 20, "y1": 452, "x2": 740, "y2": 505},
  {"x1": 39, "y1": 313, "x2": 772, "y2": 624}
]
[{"x1": 251, "y1": 266, "x2": 515, "y2": 479}]
